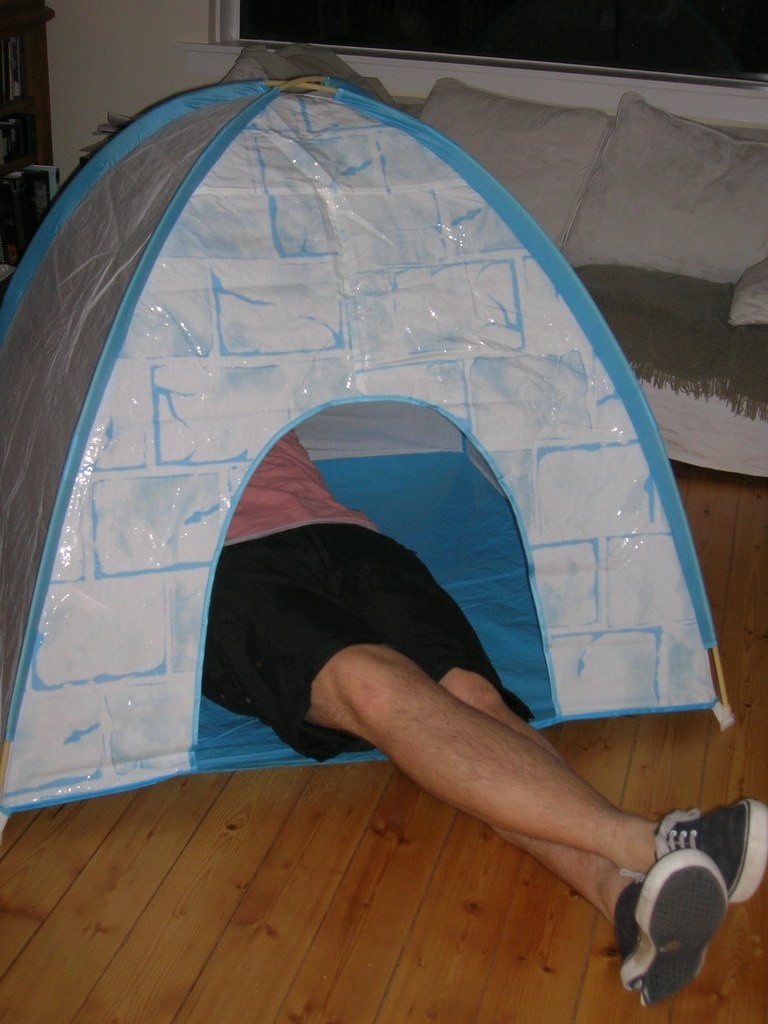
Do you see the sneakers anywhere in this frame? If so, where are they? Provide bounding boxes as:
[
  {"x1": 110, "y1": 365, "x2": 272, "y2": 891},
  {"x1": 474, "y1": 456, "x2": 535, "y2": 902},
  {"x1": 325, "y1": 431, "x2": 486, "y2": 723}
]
[
  {"x1": 614, "y1": 848, "x2": 728, "y2": 1008},
  {"x1": 653, "y1": 799, "x2": 768, "y2": 902}
]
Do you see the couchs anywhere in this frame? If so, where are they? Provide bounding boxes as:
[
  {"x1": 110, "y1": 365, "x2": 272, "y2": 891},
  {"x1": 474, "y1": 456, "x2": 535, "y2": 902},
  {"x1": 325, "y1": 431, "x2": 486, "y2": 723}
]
[{"x1": 216, "y1": 40, "x2": 768, "y2": 479}]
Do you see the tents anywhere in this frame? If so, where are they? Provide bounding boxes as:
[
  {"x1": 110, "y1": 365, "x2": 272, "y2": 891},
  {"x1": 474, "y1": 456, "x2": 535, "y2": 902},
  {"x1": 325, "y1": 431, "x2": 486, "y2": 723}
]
[{"x1": 0, "y1": 79, "x2": 732, "y2": 806}]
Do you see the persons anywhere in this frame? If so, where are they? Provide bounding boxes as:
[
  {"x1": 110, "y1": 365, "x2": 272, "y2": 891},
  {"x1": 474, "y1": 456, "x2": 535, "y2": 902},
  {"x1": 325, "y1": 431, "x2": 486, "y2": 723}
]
[{"x1": 199, "y1": 425, "x2": 768, "y2": 1007}]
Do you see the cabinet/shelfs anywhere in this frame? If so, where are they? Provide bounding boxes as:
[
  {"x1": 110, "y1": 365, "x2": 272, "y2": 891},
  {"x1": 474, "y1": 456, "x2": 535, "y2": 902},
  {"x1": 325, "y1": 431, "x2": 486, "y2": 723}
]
[{"x1": 0, "y1": 0, "x2": 56, "y2": 175}]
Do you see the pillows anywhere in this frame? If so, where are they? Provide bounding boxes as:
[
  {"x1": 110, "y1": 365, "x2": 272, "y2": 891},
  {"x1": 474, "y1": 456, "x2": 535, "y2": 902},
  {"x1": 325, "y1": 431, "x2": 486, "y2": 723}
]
[
  {"x1": 727, "y1": 256, "x2": 768, "y2": 327},
  {"x1": 418, "y1": 77, "x2": 613, "y2": 250},
  {"x1": 559, "y1": 89, "x2": 768, "y2": 285}
]
